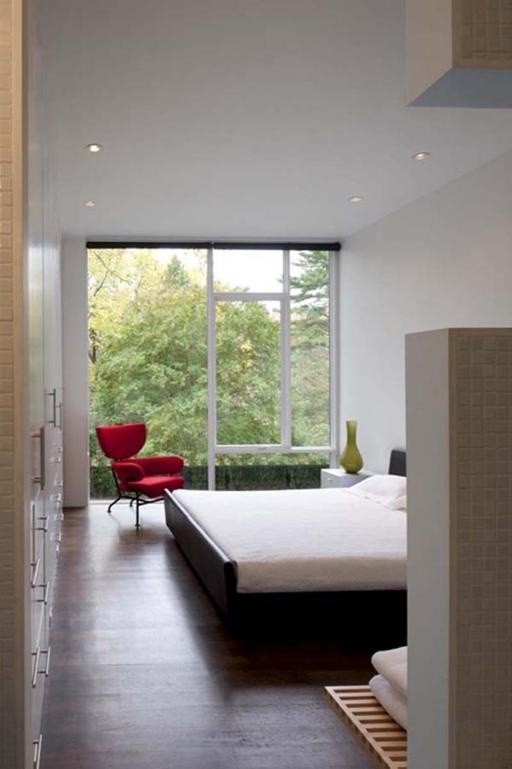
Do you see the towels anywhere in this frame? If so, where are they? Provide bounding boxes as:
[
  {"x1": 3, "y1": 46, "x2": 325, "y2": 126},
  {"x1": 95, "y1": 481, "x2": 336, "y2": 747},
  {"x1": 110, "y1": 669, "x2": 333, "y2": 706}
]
[{"x1": 369, "y1": 645, "x2": 408, "y2": 732}]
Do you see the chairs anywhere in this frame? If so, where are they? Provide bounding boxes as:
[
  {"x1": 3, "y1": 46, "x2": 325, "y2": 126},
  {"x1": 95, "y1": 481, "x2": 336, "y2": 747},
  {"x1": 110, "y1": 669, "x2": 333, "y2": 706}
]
[{"x1": 95, "y1": 421, "x2": 185, "y2": 532}]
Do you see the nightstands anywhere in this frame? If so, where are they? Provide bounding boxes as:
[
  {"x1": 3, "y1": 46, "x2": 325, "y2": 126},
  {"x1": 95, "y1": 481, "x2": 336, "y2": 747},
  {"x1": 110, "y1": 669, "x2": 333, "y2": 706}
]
[{"x1": 319, "y1": 465, "x2": 374, "y2": 490}]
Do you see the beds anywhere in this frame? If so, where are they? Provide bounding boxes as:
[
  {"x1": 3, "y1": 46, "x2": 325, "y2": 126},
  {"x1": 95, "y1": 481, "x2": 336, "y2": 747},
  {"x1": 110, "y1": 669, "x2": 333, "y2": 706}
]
[{"x1": 161, "y1": 447, "x2": 407, "y2": 657}]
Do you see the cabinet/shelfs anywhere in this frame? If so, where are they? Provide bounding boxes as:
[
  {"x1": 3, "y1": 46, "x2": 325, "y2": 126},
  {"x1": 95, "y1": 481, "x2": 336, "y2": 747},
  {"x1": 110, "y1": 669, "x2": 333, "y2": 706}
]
[{"x1": 0, "y1": 0, "x2": 65, "y2": 768}]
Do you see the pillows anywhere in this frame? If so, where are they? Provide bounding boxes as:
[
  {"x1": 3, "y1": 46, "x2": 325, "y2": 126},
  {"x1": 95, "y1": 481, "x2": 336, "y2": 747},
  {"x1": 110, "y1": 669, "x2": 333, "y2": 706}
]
[{"x1": 348, "y1": 472, "x2": 407, "y2": 511}]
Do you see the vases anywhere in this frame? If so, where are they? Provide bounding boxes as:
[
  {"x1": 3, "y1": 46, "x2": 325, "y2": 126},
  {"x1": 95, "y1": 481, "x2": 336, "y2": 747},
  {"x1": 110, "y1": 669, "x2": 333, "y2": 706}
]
[{"x1": 338, "y1": 419, "x2": 364, "y2": 474}]
